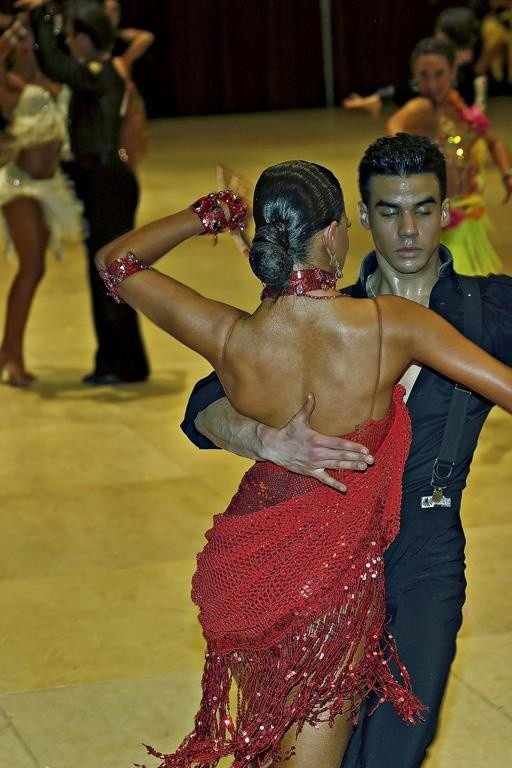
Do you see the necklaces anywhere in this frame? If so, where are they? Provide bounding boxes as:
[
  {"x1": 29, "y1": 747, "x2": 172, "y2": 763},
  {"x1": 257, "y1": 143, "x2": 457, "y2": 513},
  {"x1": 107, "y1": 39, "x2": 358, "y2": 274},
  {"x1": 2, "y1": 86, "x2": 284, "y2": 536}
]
[{"x1": 259, "y1": 269, "x2": 350, "y2": 304}]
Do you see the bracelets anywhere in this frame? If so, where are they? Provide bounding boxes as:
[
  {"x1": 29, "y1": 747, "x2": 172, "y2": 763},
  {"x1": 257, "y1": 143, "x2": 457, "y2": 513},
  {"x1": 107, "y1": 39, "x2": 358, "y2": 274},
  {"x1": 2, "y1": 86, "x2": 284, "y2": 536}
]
[
  {"x1": 189, "y1": 187, "x2": 223, "y2": 237},
  {"x1": 216, "y1": 189, "x2": 244, "y2": 233}
]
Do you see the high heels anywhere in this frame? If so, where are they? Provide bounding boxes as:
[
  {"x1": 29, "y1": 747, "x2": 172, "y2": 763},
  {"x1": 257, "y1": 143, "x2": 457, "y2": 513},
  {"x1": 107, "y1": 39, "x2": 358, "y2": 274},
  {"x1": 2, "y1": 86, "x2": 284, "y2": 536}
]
[{"x1": 1, "y1": 345, "x2": 39, "y2": 388}]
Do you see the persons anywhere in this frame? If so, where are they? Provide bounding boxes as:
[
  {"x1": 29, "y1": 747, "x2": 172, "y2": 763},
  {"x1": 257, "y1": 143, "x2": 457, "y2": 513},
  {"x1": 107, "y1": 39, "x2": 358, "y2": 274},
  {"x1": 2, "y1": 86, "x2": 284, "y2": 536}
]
[
  {"x1": 338, "y1": 7, "x2": 478, "y2": 122},
  {"x1": 385, "y1": 40, "x2": 512, "y2": 280},
  {"x1": 96, "y1": 161, "x2": 512, "y2": 768},
  {"x1": 178, "y1": 131, "x2": 512, "y2": 768},
  {"x1": 1, "y1": 0, "x2": 165, "y2": 394}
]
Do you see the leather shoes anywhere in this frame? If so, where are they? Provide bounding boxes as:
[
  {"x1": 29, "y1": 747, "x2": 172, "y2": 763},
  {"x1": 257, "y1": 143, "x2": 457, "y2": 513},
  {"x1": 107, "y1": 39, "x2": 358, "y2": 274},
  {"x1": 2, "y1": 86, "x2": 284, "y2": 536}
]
[{"x1": 83, "y1": 358, "x2": 151, "y2": 386}]
